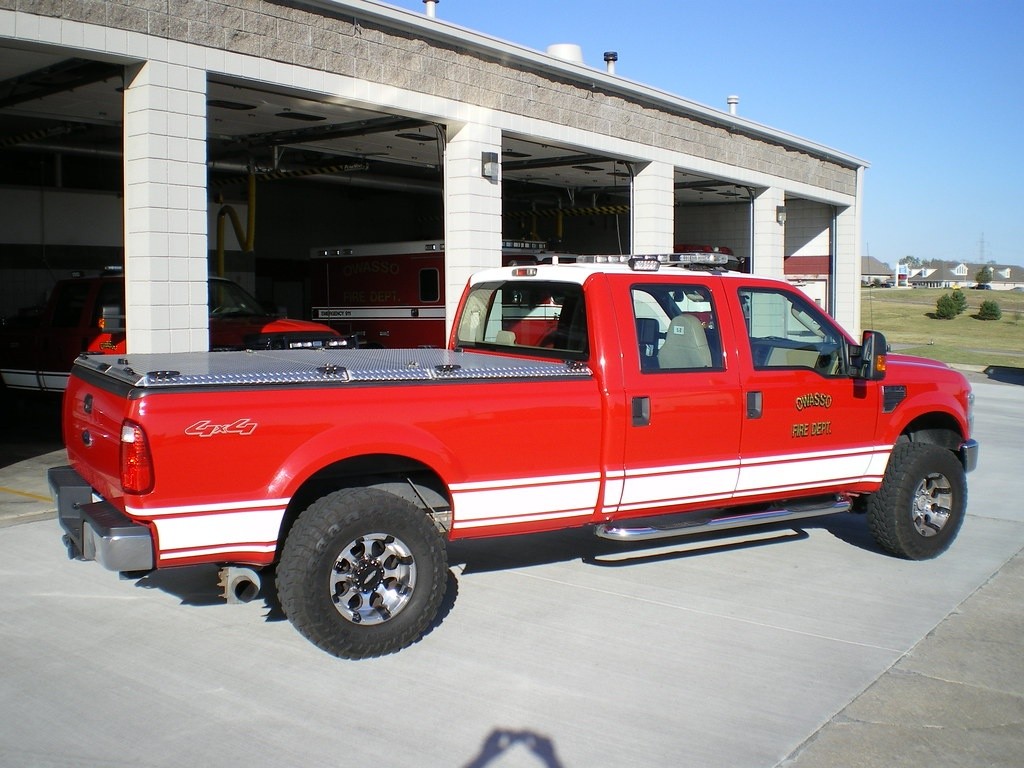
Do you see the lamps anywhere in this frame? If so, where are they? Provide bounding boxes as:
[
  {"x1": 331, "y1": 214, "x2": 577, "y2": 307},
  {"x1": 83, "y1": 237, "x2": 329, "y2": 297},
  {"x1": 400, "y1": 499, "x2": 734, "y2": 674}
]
[
  {"x1": 481, "y1": 151, "x2": 499, "y2": 177},
  {"x1": 777, "y1": 205, "x2": 787, "y2": 222}
]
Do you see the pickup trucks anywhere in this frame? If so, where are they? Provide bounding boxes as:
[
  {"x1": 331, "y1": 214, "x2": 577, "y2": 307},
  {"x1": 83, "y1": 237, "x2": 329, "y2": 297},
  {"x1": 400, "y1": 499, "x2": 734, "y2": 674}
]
[{"x1": 48, "y1": 238, "x2": 980, "y2": 662}]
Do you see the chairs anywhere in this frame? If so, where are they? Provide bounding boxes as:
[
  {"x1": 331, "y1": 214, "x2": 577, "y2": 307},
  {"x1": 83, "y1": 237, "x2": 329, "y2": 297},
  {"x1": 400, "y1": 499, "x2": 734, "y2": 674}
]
[
  {"x1": 637, "y1": 317, "x2": 658, "y2": 371},
  {"x1": 657, "y1": 313, "x2": 714, "y2": 367}
]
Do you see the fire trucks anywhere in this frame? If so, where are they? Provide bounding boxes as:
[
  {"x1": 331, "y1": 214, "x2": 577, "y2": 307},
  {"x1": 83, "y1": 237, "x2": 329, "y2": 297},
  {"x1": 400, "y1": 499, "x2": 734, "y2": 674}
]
[
  {"x1": 306, "y1": 238, "x2": 585, "y2": 363},
  {"x1": 671, "y1": 242, "x2": 748, "y2": 364},
  {"x1": 0, "y1": 264, "x2": 367, "y2": 432}
]
